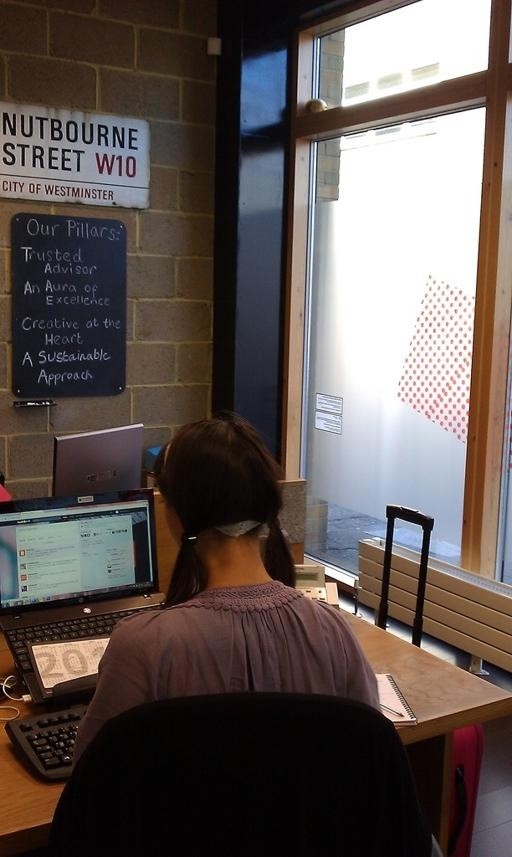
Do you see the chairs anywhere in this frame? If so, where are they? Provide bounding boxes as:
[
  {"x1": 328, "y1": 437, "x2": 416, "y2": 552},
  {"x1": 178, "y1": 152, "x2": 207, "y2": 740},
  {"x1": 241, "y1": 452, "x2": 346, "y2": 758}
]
[{"x1": 43, "y1": 689, "x2": 436, "y2": 856}]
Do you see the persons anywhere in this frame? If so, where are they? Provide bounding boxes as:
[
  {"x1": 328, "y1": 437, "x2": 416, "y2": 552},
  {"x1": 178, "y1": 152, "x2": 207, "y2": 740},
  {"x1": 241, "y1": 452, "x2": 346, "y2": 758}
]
[{"x1": 69, "y1": 408, "x2": 379, "y2": 771}]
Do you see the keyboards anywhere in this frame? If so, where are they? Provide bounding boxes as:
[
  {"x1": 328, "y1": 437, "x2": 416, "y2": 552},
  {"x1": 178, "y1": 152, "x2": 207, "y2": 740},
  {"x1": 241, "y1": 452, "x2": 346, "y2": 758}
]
[{"x1": 3, "y1": 701, "x2": 90, "y2": 785}]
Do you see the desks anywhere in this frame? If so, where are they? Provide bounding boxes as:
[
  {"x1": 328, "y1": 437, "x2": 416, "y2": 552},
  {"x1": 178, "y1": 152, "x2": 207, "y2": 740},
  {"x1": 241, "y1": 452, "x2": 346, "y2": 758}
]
[{"x1": 1, "y1": 590, "x2": 511, "y2": 856}]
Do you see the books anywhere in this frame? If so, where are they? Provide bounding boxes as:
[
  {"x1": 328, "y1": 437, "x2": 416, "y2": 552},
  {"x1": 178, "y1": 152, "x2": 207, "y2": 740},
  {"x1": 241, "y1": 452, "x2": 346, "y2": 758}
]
[{"x1": 372, "y1": 672, "x2": 419, "y2": 729}]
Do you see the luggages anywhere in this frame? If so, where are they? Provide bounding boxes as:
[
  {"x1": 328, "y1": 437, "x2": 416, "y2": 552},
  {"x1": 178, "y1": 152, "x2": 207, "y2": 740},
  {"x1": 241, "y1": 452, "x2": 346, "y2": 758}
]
[{"x1": 375, "y1": 503, "x2": 484, "y2": 855}]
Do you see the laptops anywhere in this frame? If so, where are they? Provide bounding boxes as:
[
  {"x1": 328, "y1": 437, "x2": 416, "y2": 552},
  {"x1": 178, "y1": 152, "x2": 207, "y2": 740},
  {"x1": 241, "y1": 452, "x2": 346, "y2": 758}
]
[
  {"x1": 1, "y1": 486, "x2": 166, "y2": 706},
  {"x1": 52, "y1": 421, "x2": 146, "y2": 499}
]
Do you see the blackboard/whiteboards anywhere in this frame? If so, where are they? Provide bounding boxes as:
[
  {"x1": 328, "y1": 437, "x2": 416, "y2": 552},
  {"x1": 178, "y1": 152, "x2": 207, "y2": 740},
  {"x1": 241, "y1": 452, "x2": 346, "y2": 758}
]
[{"x1": 10, "y1": 212, "x2": 127, "y2": 398}]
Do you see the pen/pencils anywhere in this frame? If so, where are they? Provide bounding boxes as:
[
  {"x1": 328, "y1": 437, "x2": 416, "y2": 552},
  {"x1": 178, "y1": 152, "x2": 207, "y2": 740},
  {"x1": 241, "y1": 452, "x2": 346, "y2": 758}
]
[{"x1": 379, "y1": 704, "x2": 404, "y2": 717}]
